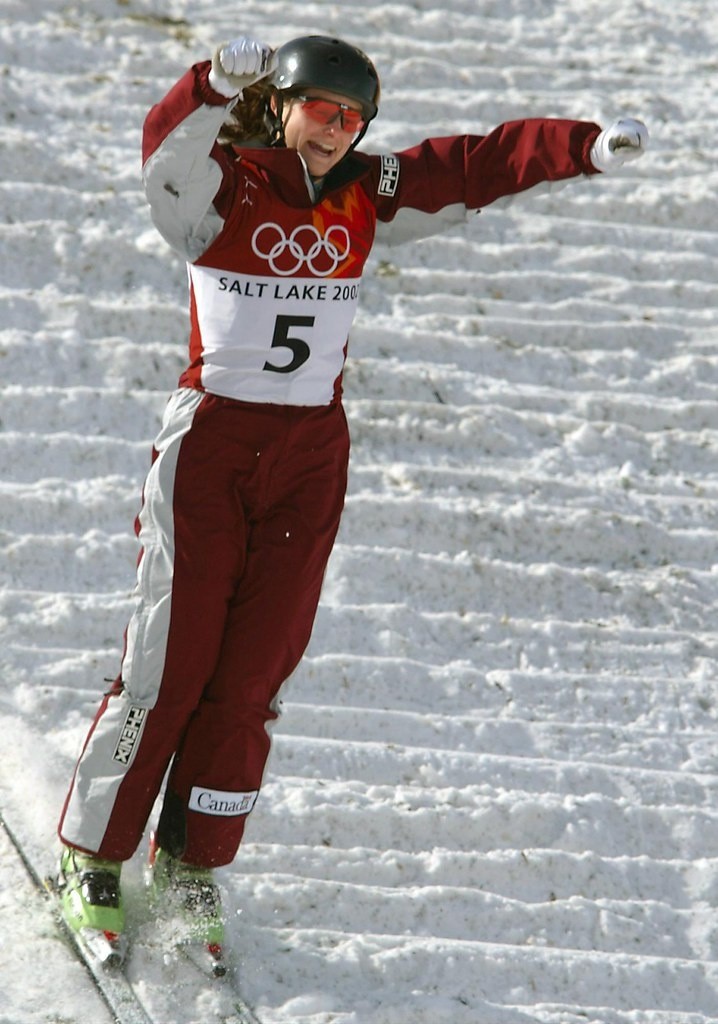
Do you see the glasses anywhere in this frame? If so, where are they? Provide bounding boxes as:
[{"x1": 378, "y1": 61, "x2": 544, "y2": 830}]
[{"x1": 283, "y1": 89, "x2": 363, "y2": 133}]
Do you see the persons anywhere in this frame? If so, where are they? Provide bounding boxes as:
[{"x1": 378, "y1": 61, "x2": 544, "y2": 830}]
[{"x1": 53, "y1": 34, "x2": 651, "y2": 955}]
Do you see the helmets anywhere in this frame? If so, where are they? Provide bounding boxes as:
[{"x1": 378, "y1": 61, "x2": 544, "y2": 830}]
[{"x1": 263, "y1": 35, "x2": 380, "y2": 122}]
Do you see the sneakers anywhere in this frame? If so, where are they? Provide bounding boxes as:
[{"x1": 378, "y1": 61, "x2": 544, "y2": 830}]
[
  {"x1": 59, "y1": 842, "x2": 121, "y2": 929},
  {"x1": 150, "y1": 846, "x2": 223, "y2": 944}
]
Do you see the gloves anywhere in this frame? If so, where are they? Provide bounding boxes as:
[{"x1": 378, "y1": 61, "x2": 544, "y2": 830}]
[
  {"x1": 208, "y1": 39, "x2": 277, "y2": 98},
  {"x1": 588, "y1": 120, "x2": 649, "y2": 174}
]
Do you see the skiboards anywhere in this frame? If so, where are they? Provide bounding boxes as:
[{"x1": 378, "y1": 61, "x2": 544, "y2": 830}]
[{"x1": 1, "y1": 807, "x2": 259, "y2": 1024}]
